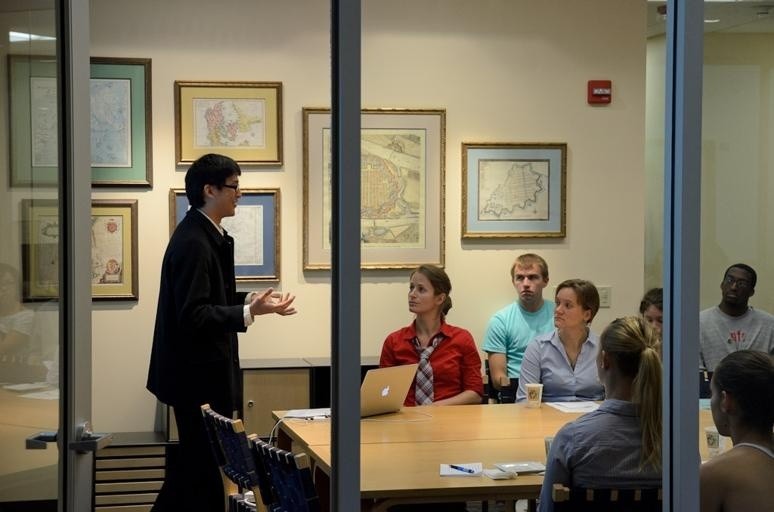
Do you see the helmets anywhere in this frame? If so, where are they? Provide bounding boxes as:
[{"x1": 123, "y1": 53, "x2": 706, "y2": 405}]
[{"x1": 494, "y1": 460, "x2": 546, "y2": 473}]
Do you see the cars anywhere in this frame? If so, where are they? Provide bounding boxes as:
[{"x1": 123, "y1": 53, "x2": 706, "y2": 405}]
[
  {"x1": 524, "y1": 383, "x2": 544, "y2": 410},
  {"x1": 704, "y1": 425, "x2": 728, "y2": 460},
  {"x1": 544, "y1": 436, "x2": 554, "y2": 459}
]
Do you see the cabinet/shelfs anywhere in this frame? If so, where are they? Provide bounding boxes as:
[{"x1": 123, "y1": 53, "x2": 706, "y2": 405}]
[{"x1": 92, "y1": 368, "x2": 312, "y2": 511}]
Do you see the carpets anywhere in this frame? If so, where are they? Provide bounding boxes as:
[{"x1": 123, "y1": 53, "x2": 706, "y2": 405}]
[{"x1": 412, "y1": 333, "x2": 445, "y2": 406}]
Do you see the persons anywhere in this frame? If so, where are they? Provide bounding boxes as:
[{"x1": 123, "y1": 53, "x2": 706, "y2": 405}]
[
  {"x1": 534, "y1": 313, "x2": 703, "y2": 511},
  {"x1": 481, "y1": 252, "x2": 559, "y2": 404},
  {"x1": 142, "y1": 151, "x2": 298, "y2": 511},
  {"x1": 0, "y1": 262, "x2": 47, "y2": 387},
  {"x1": 698, "y1": 349, "x2": 774, "y2": 510},
  {"x1": 698, "y1": 261, "x2": 774, "y2": 398},
  {"x1": 637, "y1": 288, "x2": 663, "y2": 338},
  {"x1": 513, "y1": 276, "x2": 607, "y2": 400},
  {"x1": 378, "y1": 262, "x2": 485, "y2": 408}
]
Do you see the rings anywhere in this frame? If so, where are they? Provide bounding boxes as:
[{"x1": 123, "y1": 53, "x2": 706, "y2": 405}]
[
  {"x1": 305, "y1": 416, "x2": 328, "y2": 420},
  {"x1": 449, "y1": 464, "x2": 475, "y2": 473}
]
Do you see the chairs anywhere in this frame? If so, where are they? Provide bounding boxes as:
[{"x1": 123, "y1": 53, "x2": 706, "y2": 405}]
[
  {"x1": 189, "y1": 401, "x2": 318, "y2": 512},
  {"x1": 476, "y1": 358, "x2": 662, "y2": 511}
]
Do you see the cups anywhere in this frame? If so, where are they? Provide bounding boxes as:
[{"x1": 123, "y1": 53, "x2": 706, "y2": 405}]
[{"x1": 224, "y1": 185, "x2": 239, "y2": 193}]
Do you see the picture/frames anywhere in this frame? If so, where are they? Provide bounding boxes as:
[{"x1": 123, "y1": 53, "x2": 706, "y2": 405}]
[
  {"x1": 302, "y1": 108, "x2": 446, "y2": 271},
  {"x1": 170, "y1": 188, "x2": 281, "y2": 283},
  {"x1": 21, "y1": 198, "x2": 139, "y2": 303},
  {"x1": 461, "y1": 142, "x2": 567, "y2": 240},
  {"x1": 9, "y1": 56, "x2": 153, "y2": 188},
  {"x1": 174, "y1": 79, "x2": 283, "y2": 168}
]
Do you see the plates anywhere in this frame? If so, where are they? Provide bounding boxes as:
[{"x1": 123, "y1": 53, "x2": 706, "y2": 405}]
[{"x1": 92, "y1": 368, "x2": 312, "y2": 511}]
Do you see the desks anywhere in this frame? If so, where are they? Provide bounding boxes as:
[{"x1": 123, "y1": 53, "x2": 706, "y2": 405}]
[{"x1": 270, "y1": 394, "x2": 773, "y2": 512}]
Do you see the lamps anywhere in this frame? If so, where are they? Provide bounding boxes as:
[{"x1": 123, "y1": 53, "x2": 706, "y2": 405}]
[
  {"x1": 189, "y1": 401, "x2": 318, "y2": 512},
  {"x1": 476, "y1": 358, "x2": 662, "y2": 511}
]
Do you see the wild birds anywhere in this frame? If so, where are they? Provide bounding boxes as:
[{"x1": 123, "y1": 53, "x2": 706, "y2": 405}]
[{"x1": 360, "y1": 363, "x2": 420, "y2": 418}]
